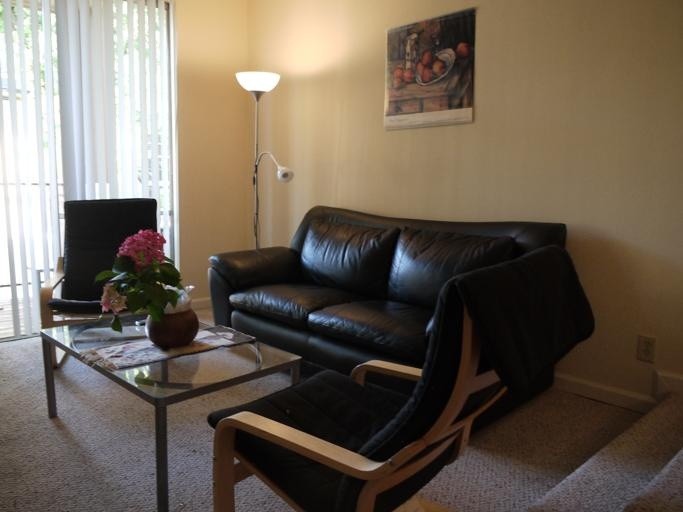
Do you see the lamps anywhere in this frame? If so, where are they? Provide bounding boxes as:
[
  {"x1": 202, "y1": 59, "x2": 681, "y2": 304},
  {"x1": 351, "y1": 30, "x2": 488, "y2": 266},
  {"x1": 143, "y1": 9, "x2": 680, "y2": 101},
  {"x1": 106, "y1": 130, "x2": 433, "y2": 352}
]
[{"x1": 235, "y1": 72, "x2": 293, "y2": 245}]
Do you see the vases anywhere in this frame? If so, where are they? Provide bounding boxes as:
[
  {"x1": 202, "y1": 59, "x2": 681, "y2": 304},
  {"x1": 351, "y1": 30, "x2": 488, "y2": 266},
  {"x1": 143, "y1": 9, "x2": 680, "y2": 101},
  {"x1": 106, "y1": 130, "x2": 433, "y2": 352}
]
[{"x1": 146, "y1": 310, "x2": 199, "y2": 351}]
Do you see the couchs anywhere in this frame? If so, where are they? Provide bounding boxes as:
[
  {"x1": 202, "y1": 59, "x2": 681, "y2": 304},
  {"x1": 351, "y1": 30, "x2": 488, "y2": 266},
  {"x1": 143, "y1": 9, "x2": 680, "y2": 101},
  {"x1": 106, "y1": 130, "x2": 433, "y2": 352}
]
[{"x1": 210, "y1": 205, "x2": 567, "y2": 388}]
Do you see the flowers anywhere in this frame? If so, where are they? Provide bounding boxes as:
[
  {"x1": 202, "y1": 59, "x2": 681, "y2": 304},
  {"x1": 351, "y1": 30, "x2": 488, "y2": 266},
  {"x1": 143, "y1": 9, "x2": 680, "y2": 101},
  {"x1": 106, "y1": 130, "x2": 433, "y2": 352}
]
[{"x1": 101, "y1": 231, "x2": 183, "y2": 332}]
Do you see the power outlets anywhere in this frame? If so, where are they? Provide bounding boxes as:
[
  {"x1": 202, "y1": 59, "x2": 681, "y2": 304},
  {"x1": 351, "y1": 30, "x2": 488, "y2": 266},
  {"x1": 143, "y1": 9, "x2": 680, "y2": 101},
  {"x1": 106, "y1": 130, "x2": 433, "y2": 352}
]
[{"x1": 636, "y1": 334, "x2": 656, "y2": 363}]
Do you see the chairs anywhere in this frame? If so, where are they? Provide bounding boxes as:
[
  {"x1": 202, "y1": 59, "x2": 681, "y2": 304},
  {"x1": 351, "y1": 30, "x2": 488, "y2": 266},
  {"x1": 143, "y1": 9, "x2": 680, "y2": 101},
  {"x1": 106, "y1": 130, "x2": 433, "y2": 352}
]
[
  {"x1": 207, "y1": 242, "x2": 596, "y2": 512},
  {"x1": 37, "y1": 197, "x2": 157, "y2": 371}
]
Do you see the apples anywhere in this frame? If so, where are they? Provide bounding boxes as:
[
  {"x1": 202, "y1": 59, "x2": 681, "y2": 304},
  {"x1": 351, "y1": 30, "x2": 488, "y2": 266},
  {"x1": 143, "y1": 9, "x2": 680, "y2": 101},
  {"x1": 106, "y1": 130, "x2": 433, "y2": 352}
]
[
  {"x1": 401, "y1": 70, "x2": 414, "y2": 83},
  {"x1": 416, "y1": 63, "x2": 425, "y2": 76},
  {"x1": 432, "y1": 60, "x2": 446, "y2": 77},
  {"x1": 393, "y1": 68, "x2": 401, "y2": 78},
  {"x1": 421, "y1": 50, "x2": 436, "y2": 66},
  {"x1": 456, "y1": 42, "x2": 471, "y2": 60},
  {"x1": 421, "y1": 68, "x2": 432, "y2": 83},
  {"x1": 393, "y1": 78, "x2": 401, "y2": 88}
]
